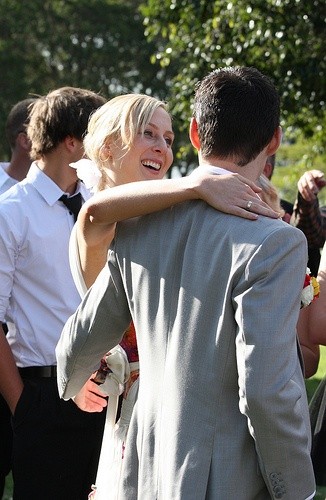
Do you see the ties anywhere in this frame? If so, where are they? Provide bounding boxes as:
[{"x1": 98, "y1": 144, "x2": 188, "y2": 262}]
[{"x1": 60, "y1": 195, "x2": 82, "y2": 221}]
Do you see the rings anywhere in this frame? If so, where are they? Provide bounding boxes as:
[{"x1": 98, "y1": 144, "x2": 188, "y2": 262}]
[{"x1": 246, "y1": 200, "x2": 253, "y2": 210}]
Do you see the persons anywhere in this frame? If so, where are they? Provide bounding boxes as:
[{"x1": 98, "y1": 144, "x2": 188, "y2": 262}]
[
  {"x1": 0, "y1": 96, "x2": 42, "y2": 500},
  {"x1": 68, "y1": 94, "x2": 285, "y2": 500},
  {"x1": 0, "y1": 88, "x2": 111, "y2": 500},
  {"x1": 58, "y1": 66, "x2": 317, "y2": 500},
  {"x1": 256, "y1": 145, "x2": 326, "y2": 383}
]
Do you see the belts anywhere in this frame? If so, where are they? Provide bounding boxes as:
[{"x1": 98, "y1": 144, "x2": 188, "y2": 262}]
[{"x1": 18, "y1": 364, "x2": 58, "y2": 380}]
